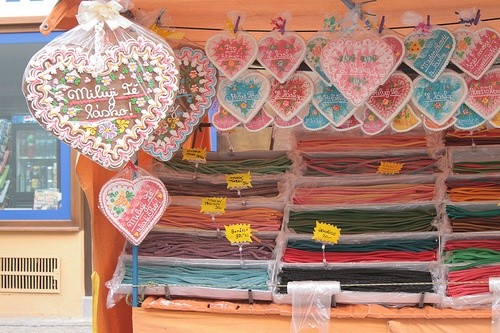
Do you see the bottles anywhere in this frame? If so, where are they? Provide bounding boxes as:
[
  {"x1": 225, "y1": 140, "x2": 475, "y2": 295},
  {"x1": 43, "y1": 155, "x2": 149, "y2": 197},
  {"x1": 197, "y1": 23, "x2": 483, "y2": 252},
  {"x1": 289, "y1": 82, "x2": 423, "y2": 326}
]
[{"x1": 20, "y1": 131, "x2": 57, "y2": 192}]
